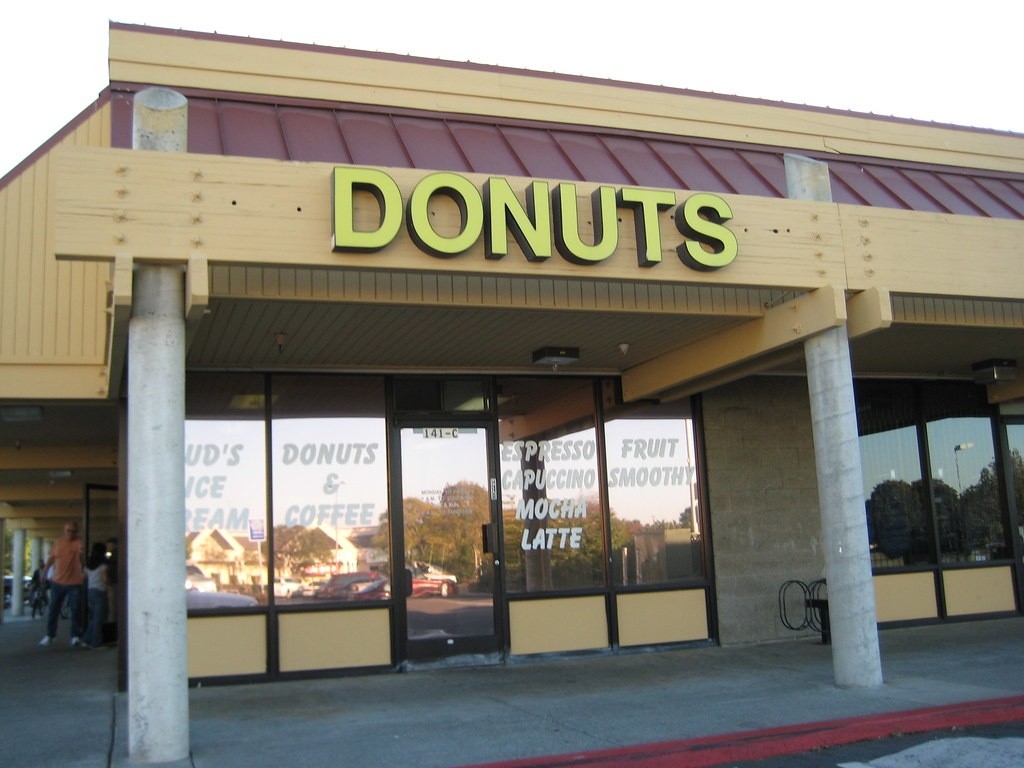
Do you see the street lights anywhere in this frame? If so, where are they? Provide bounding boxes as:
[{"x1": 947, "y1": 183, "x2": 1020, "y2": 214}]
[
  {"x1": 328, "y1": 479, "x2": 347, "y2": 576},
  {"x1": 953, "y1": 442, "x2": 975, "y2": 553}
]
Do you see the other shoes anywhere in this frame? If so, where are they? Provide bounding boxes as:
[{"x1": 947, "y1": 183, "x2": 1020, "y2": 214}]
[
  {"x1": 39, "y1": 635, "x2": 51, "y2": 647},
  {"x1": 81, "y1": 642, "x2": 93, "y2": 649},
  {"x1": 71, "y1": 636, "x2": 81, "y2": 647},
  {"x1": 92, "y1": 646, "x2": 110, "y2": 650}
]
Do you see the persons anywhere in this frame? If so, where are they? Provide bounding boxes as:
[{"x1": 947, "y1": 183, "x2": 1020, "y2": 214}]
[
  {"x1": 82, "y1": 536, "x2": 121, "y2": 651},
  {"x1": 27, "y1": 560, "x2": 48, "y2": 621},
  {"x1": 38, "y1": 520, "x2": 86, "y2": 645}
]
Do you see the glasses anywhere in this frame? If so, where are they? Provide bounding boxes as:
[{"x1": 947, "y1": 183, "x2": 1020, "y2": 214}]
[{"x1": 66, "y1": 529, "x2": 77, "y2": 534}]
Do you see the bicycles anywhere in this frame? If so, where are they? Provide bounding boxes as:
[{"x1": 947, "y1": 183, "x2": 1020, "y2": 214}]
[
  {"x1": 27, "y1": 582, "x2": 48, "y2": 617},
  {"x1": 41, "y1": 579, "x2": 70, "y2": 619}
]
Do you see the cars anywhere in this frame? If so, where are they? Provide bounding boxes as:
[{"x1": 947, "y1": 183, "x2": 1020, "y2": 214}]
[
  {"x1": 4, "y1": 574, "x2": 35, "y2": 608},
  {"x1": 302, "y1": 557, "x2": 457, "y2": 601},
  {"x1": 264, "y1": 577, "x2": 304, "y2": 599},
  {"x1": 186, "y1": 587, "x2": 258, "y2": 610}
]
